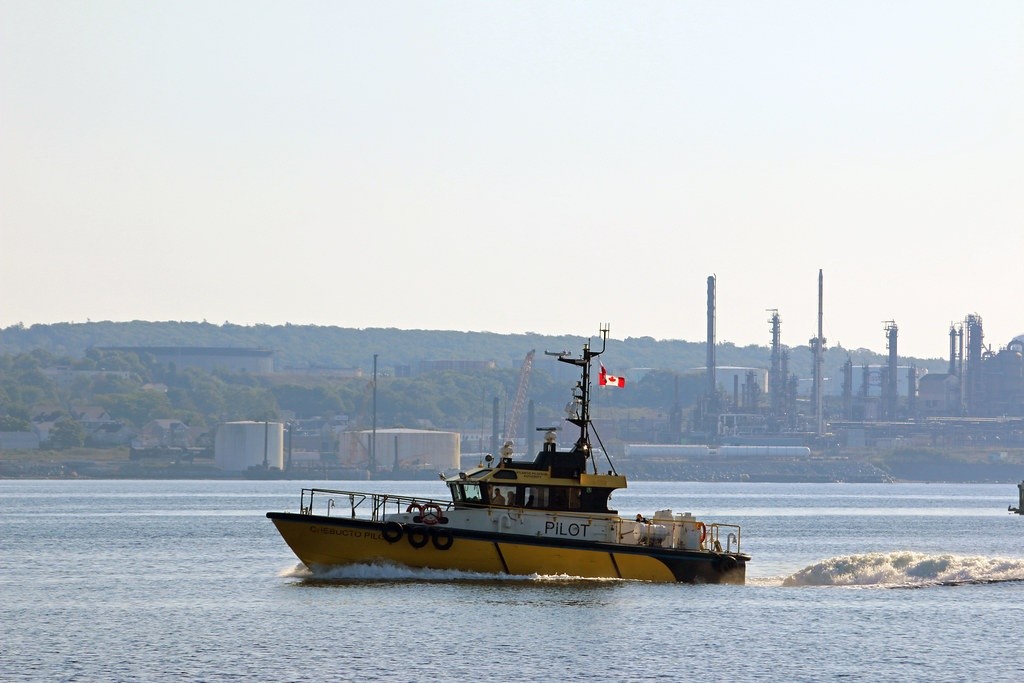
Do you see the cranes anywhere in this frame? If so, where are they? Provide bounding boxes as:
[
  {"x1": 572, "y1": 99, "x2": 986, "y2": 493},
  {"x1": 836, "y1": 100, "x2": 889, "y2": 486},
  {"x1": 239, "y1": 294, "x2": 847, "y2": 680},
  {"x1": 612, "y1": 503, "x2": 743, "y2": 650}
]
[{"x1": 501, "y1": 347, "x2": 536, "y2": 449}]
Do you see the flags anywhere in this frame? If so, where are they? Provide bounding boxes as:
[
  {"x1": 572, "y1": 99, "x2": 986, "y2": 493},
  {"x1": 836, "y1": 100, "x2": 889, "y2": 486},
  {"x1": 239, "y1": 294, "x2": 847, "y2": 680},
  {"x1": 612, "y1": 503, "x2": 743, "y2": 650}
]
[{"x1": 598, "y1": 361, "x2": 625, "y2": 388}]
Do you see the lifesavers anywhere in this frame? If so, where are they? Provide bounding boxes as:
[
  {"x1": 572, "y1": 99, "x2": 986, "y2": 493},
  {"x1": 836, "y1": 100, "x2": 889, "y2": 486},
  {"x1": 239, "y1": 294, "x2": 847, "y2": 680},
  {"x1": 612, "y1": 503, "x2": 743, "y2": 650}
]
[
  {"x1": 381, "y1": 520, "x2": 454, "y2": 551},
  {"x1": 698, "y1": 522, "x2": 707, "y2": 543},
  {"x1": 406, "y1": 502, "x2": 443, "y2": 525}
]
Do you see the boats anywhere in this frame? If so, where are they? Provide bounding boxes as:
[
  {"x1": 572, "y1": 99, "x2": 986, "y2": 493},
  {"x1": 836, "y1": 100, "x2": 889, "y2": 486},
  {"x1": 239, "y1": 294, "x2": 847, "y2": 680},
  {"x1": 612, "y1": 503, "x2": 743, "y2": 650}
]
[{"x1": 263, "y1": 340, "x2": 751, "y2": 583}]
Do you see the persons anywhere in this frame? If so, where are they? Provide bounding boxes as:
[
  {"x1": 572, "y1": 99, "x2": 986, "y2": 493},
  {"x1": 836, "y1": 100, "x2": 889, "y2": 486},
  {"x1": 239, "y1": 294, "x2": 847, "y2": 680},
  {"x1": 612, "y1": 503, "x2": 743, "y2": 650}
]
[
  {"x1": 636, "y1": 513, "x2": 647, "y2": 524},
  {"x1": 492, "y1": 488, "x2": 515, "y2": 506}
]
